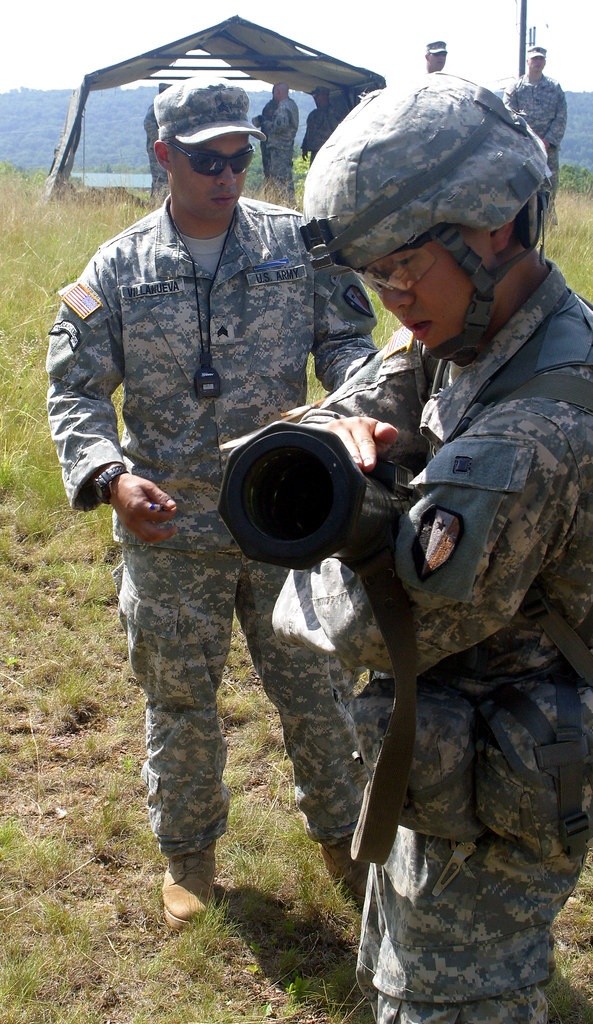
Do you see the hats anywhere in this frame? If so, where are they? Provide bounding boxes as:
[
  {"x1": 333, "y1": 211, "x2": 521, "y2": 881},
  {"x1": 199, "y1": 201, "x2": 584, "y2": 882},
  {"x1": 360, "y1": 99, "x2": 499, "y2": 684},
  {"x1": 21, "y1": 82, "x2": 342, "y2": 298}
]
[
  {"x1": 153, "y1": 75, "x2": 267, "y2": 145},
  {"x1": 311, "y1": 86, "x2": 329, "y2": 95},
  {"x1": 526, "y1": 47, "x2": 546, "y2": 59},
  {"x1": 427, "y1": 41, "x2": 447, "y2": 53}
]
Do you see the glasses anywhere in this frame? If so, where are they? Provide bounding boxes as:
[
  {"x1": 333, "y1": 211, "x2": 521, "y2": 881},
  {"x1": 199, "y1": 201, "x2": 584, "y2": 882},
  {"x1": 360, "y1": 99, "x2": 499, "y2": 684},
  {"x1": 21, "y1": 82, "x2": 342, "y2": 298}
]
[
  {"x1": 355, "y1": 240, "x2": 443, "y2": 295},
  {"x1": 161, "y1": 139, "x2": 253, "y2": 176}
]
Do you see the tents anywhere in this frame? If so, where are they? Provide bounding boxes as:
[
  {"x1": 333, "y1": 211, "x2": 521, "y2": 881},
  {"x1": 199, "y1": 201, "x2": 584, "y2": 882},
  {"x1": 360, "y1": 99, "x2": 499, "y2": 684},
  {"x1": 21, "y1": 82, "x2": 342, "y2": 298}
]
[{"x1": 45, "y1": 15, "x2": 387, "y2": 198}]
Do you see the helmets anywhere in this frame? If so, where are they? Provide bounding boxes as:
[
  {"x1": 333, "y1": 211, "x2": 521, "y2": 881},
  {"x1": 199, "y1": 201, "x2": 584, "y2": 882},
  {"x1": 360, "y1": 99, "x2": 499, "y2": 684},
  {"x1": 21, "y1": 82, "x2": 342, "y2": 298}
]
[{"x1": 302, "y1": 72, "x2": 546, "y2": 268}]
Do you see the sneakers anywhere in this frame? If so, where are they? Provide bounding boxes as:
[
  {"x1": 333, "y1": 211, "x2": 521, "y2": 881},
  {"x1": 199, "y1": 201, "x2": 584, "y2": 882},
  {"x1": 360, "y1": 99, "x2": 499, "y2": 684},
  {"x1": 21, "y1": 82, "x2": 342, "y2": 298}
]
[
  {"x1": 161, "y1": 839, "x2": 216, "y2": 928},
  {"x1": 318, "y1": 843, "x2": 380, "y2": 904}
]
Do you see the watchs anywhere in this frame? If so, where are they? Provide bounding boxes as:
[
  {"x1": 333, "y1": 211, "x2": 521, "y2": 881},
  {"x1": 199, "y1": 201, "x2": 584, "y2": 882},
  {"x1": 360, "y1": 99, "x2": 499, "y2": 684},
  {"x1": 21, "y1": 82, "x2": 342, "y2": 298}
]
[{"x1": 93, "y1": 466, "x2": 129, "y2": 504}]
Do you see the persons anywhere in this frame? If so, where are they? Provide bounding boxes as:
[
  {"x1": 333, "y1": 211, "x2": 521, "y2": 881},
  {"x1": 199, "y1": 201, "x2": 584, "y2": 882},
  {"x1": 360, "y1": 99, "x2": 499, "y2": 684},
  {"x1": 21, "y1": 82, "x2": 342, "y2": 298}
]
[
  {"x1": 46, "y1": 76, "x2": 379, "y2": 929},
  {"x1": 301, "y1": 86, "x2": 343, "y2": 169},
  {"x1": 271, "y1": 73, "x2": 593, "y2": 1024},
  {"x1": 143, "y1": 83, "x2": 173, "y2": 200},
  {"x1": 251, "y1": 83, "x2": 299, "y2": 206},
  {"x1": 501, "y1": 47, "x2": 567, "y2": 227},
  {"x1": 425, "y1": 40, "x2": 447, "y2": 73}
]
